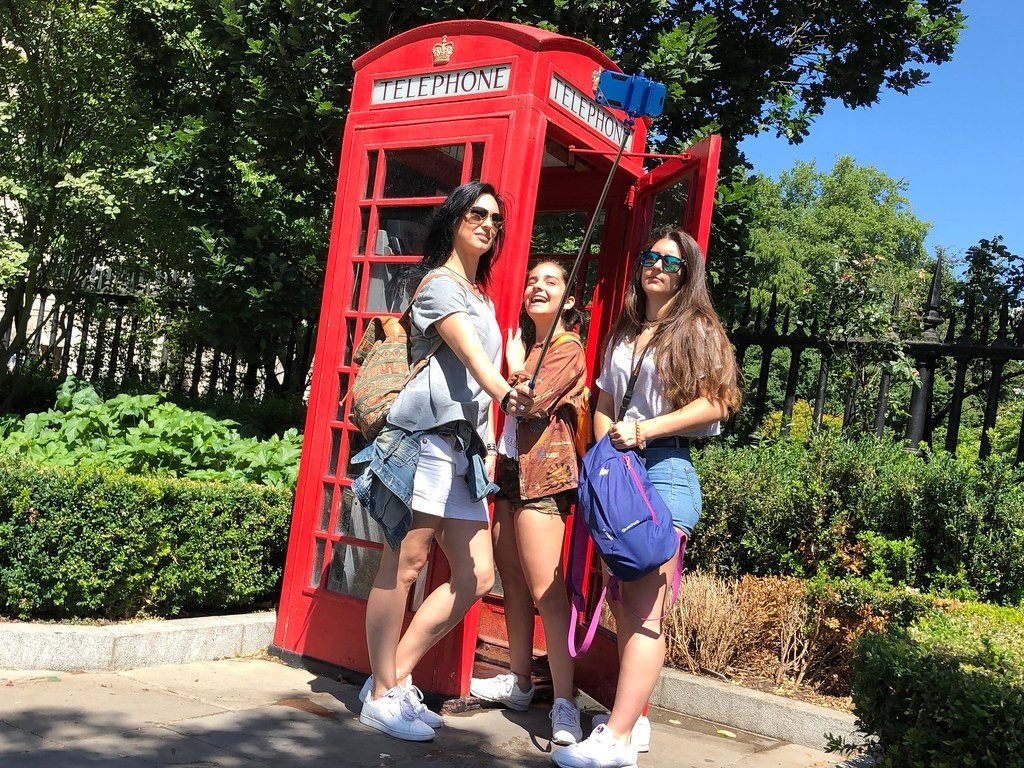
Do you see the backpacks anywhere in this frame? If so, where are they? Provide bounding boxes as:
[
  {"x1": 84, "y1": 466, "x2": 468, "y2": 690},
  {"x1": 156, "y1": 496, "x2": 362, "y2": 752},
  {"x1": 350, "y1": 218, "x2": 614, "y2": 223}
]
[
  {"x1": 568, "y1": 429, "x2": 686, "y2": 659},
  {"x1": 548, "y1": 335, "x2": 594, "y2": 459},
  {"x1": 339, "y1": 274, "x2": 467, "y2": 443}
]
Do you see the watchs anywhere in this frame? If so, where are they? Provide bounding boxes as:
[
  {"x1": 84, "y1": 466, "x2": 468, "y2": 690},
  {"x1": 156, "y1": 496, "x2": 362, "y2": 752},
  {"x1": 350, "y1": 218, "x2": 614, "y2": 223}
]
[{"x1": 500, "y1": 390, "x2": 514, "y2": 415}]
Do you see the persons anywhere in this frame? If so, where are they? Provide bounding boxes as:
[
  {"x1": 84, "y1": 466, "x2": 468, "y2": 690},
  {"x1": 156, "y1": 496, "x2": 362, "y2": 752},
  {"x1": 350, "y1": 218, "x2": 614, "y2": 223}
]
[
  {"x1": 550, "y1": 229, "x2": 742, "y2": 768},
  {"x1": 351, "y1": 180, "x2": 532, "y2": 742},
  {"x1": 471, "y1": 260, "x2": 587, "y2": 745}
]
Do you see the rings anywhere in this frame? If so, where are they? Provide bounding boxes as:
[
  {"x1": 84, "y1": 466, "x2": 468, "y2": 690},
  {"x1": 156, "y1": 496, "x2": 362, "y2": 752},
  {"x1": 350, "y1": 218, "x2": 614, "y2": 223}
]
[{"x1": 518, "y1": 404, "x2": 525, "y2": 413}]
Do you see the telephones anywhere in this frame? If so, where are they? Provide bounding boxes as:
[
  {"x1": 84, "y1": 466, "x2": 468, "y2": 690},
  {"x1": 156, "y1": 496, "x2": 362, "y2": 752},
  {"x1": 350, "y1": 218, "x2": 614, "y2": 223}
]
[{"x1": 360, "y1": 218, "x2": 436, "y2": 314}]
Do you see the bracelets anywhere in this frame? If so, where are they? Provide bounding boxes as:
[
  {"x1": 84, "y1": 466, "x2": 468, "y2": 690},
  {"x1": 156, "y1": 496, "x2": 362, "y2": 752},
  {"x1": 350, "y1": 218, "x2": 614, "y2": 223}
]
[{"x1": 635, "y1": 421, "x2": 646, "y2": 450}]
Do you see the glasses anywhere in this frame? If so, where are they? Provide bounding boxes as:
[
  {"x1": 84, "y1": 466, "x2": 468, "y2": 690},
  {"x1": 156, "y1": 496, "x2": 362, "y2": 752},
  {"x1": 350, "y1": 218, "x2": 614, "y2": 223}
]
[
  {"x1": 640, "y1": 250, "x2": 688, "y2": 273},
  {"x1": 467, "y1": 206, "x2": 504, "y2": 230}
]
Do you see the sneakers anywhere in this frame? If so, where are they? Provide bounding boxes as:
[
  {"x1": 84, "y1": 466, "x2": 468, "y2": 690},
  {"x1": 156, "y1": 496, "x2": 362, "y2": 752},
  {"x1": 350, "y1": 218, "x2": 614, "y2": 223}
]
[
  {"x1": 359, "y1": 674, "x2": 441, "y2": 741},
  {"x1": 469, "y1": 671, "x2": 535, "y2": 711},
  {"x1": 592, "y1": 714, "x2": 651, "y2": 752},
  {"x1": 551, "y1": 723, "x2": 638, "y2": 768},
  {"x1": 549, "y1": 698, "x2": 582, "y2": 745}
]
